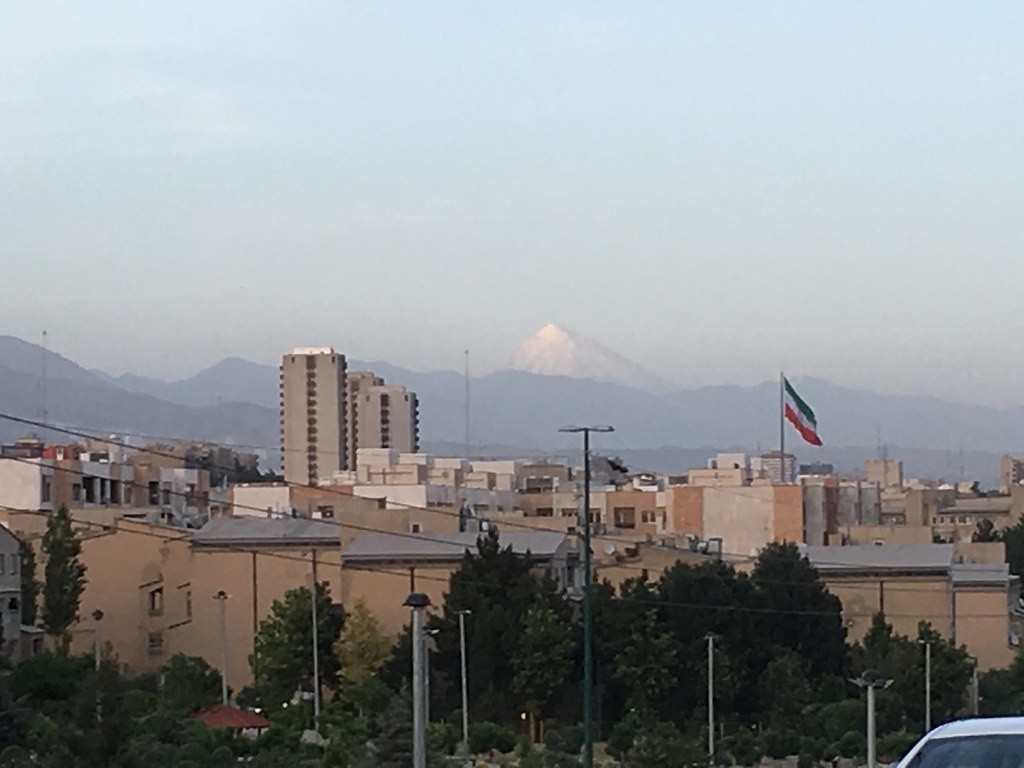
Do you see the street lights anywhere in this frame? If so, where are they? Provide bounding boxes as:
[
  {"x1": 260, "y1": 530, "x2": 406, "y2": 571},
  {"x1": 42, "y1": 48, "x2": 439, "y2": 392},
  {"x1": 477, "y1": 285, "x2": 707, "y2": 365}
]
[
  {"x1": 703, "y1": 631, "x2": 724, "y2": 756},
  {"x1": 402, "y1": 592, "x2": 432, "y2": 768},
  {"x1": 559, "y1": 425, "x2": 615, "y2": 768},
  {"x1": 449, "y1": 598, "x2": 474, "y2": 745},
  {"x1": 212, "y1": 590, "x2": 233, "y2": 705},
  {"x1": 916, "y1": 632, "x2": 939, "y2": 735},
  {"x1": 849, "y1": 669, "x2": 894, "y2": 767},
  {"x1": 91, "y1": 609, "x2": 104, "y2": 722}
]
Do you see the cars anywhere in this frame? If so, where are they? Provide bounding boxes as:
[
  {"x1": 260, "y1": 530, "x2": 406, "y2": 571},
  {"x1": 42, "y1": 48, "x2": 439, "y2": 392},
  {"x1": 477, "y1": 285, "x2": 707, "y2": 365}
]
[
  {"x1": 235, "y1": 670, "x2": 378, "y2": 739},
  {"x1": 894, "y1": 715, "x2": 1024, "y2": 768}
]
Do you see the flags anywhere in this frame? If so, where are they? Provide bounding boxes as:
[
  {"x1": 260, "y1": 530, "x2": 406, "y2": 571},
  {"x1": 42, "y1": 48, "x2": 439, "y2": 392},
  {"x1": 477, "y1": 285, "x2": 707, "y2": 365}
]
[{"x1": 782, "y1": 376, "x2": 822, "y2": 446}]
